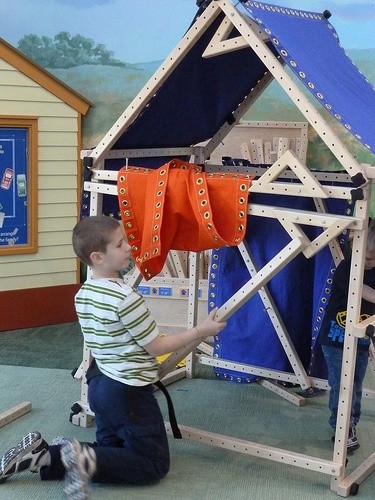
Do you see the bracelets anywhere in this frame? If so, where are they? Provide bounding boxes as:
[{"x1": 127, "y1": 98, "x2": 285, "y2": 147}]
[{"x1": 195, "y1": 325, "x2": 203, "y2": 339}]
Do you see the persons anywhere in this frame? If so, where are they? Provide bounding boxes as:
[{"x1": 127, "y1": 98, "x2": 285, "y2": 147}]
[
  {"x1": 317, "y1": 216, "x2": 375, "y2": 464},
  {"x1": 0, "y1": 215, "x2": 227, "y2": 500}
]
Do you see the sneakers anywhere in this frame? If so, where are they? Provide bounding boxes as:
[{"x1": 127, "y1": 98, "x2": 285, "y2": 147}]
[
  {"x1": 347, "y1": 424, "x2": 361, "y2": 453},
  {"x1": 331, "y1": 428, "x2": 335, "y2": 442},
  {"x1": 0, "y1": 431, "x2": 49, "y2": 485},
  {"x1": 60, "y1": 438, "x2": 98, "y2": 500}
]
